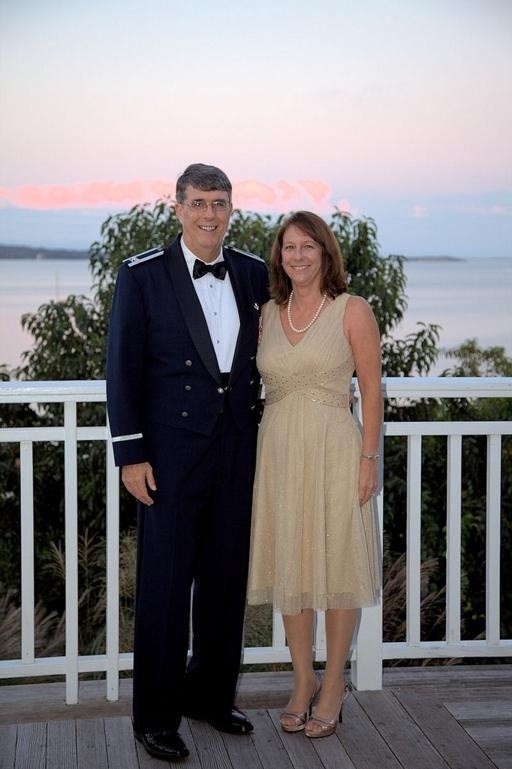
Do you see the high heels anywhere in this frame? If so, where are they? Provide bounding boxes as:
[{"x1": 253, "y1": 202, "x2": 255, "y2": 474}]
[
  {"x1": 278, "y1": 673, "x2": 322, "y2": 734},
  {"x1": 303, "y1": 680, "x2": 355, "y2": 740}
]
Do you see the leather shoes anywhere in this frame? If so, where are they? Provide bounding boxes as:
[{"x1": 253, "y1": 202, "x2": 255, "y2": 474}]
[
  {"x1": 205, "y1": 707, "x2": 256, "y2": 736},
  {"x1": 132, "y1": 729, "x2": 190, "y2": 760}
]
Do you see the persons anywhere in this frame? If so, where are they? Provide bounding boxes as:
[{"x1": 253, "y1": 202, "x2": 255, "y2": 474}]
[
  {"x1": 104, "y1": 162, "x2": 361, "y2": 765},
  {"x1": 255, "y1": 208, "x2": 386, "y2": 744}
]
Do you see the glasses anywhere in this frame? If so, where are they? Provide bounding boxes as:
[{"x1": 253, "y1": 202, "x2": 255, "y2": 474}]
[{"x1": 180, "y1": 199, "x2": 232, "y2": 212}]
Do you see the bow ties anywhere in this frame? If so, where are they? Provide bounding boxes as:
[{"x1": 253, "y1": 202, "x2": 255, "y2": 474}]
[{"x1": 192, "y1": 257, "x2": 229, "y2": 282}]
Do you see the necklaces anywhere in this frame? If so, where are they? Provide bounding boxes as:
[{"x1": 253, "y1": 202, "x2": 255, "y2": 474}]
[{"x1": 285, "y1": 290, "x2": 329, "y2": 334}]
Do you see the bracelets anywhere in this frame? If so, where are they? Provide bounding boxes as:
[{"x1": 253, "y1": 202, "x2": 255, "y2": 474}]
[{"x1": 361, "y1": 450, "x2": 383, "y2": 463}]
